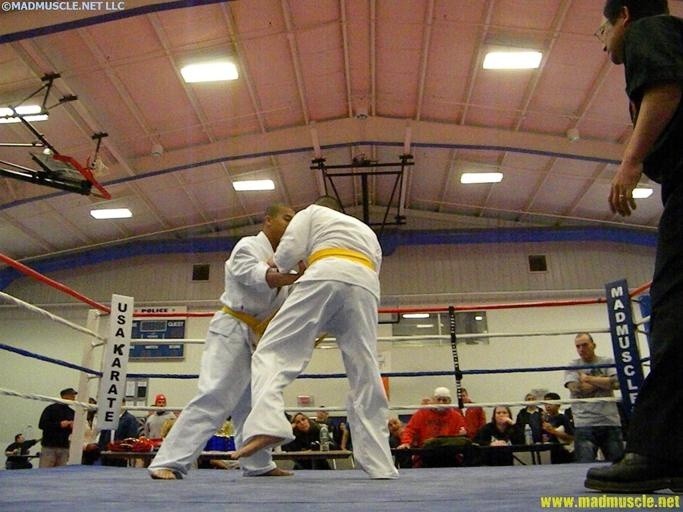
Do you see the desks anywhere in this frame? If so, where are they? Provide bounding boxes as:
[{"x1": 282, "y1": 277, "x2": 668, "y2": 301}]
[
  {"x1": 100, "y1": 451, "x2": 352, "y2": 470},
  {"x1": 391, "y1": 443, "x2": 569, "y2": 469}
]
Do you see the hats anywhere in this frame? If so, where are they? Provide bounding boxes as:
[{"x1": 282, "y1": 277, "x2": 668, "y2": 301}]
[
  {"x1": 544, "y1": 391, "x2": 561, "y2": 399},
  {"x1": 433, "y1": 387, "x2": 453, "y2": 402},
  {"x1": 525, "y1": 393, "x2": 535, "y2": 402},
  {"x1": 60, "y1": 388, "x2": 78, "y2": 395}
]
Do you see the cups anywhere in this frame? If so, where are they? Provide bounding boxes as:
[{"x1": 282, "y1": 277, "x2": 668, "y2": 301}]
[{"x1": 16, "y1": 448, "x2": 21, "y2": 456}]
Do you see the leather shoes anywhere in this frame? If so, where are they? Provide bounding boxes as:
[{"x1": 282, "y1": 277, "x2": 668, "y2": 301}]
[{"x1": 583, "y1": 451, "x2": 683, "y2": 495}]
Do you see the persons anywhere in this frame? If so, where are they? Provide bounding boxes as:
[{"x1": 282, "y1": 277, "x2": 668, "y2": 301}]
[
  {"x1": 82, "y1": 391, "x2": 184, "y2": 467},
  {"x1": 146, "y1": 201, "x2": 306, "y2": 478},
  {"x1": 38, "y1": 388, "x2": 78, "y2": 467},
  {"x1": 583, "y1": 1, "x2": 679, "y2": 493},
  {"x1": 388, "y1": 387, "x2": 578, "y2": 469},
  {"x1": 563, "y1": 332, "x2": 624, "y2": 465},
  {"x1": 283, "y1": 405, "x2": 355, "y2": 470},
  {"x1": 230, "y1": 196, "x2": 400, "y2": 478},
  {"x1": 5, "y1": 434, "x2": 40, "y2": 469}
]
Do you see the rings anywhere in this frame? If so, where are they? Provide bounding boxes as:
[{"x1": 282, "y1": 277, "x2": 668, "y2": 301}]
[{"x1": 618, "y1": 194, "x2": 625, "y2": 198}]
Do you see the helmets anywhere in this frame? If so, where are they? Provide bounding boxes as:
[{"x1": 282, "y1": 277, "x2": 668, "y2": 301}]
[{"x1": 155, "y1": 394, "x2": 166, "y2": 401}]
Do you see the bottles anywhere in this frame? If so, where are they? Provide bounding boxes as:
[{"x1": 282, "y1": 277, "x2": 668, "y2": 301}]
[
  {"x1": 524, "y1": 424, "x2": 533, "y2": 445},
  {"x1": 320, "y1": 424, "x2": 329, "y2": 451}
]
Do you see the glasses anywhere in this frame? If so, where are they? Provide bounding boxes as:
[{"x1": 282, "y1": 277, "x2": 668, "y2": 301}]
[
  {"x1": 436, "y1": 398, "x2": 450, "y2": 402},
  {"x1": 594, "y1": 19, "x2": 607, "y2": 44}
]
